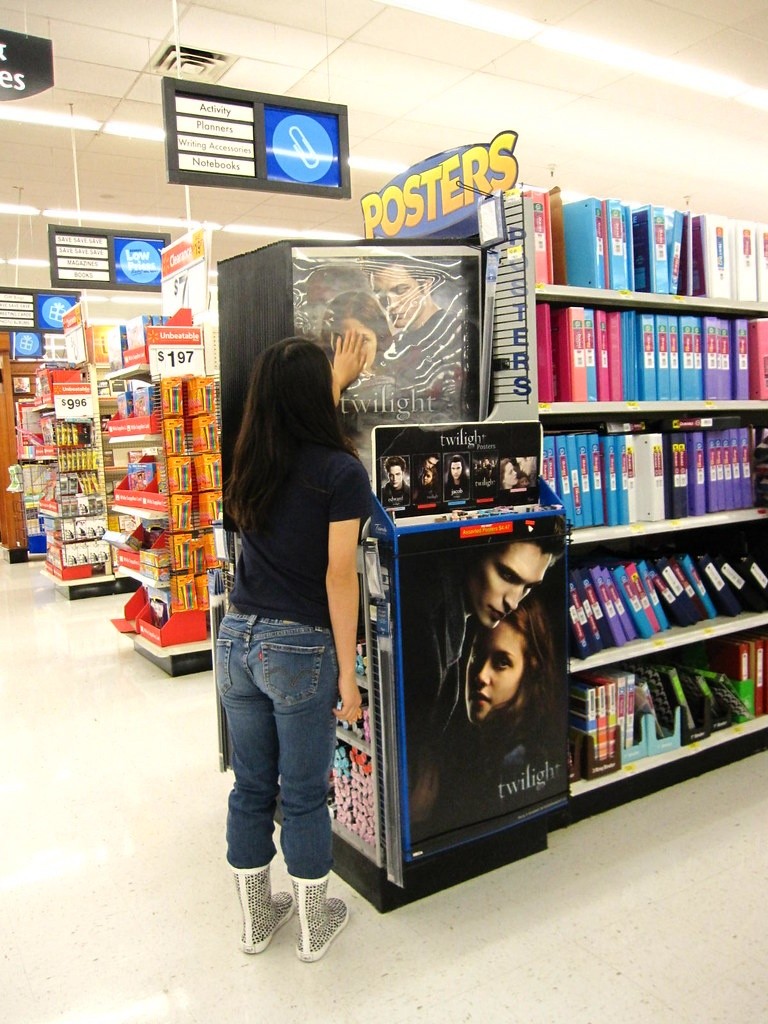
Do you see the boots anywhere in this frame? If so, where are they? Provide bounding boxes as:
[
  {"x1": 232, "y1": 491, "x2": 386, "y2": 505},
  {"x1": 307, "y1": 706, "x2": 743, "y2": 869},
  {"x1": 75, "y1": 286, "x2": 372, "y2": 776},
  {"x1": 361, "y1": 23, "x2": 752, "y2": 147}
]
[
  {"x1": 289, "y1": 873, "x2": 349, "y2": 963},
  {"x1": 229, "y1": 862, "x2": 295, "y2": 954}
]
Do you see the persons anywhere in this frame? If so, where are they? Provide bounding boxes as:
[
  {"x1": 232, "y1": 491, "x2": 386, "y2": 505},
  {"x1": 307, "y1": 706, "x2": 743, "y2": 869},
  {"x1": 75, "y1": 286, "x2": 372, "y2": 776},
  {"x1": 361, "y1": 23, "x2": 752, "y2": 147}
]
[
  {"x1": 213, "y1": 330, "x2": 370, "y2": 963},
  {"x1": 313, "y1": 253, "x2": 479, "y2": 420},
  {"x1": 372, "y1": 450, "x2": 563, "y2": 832}
]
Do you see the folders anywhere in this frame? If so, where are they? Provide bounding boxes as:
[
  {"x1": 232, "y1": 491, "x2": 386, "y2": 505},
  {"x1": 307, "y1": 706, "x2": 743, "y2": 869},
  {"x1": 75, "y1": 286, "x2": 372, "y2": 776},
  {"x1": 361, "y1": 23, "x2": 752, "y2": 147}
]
[
  {"x1": 530, "y1": 187, "x2": 768, "y2": 532},
  {"x1": 657, "y1": 622, "x2": 768, "y2": 731},
  {"x1": 567, "y1": 553, "x2": 768, "y2": 662}
]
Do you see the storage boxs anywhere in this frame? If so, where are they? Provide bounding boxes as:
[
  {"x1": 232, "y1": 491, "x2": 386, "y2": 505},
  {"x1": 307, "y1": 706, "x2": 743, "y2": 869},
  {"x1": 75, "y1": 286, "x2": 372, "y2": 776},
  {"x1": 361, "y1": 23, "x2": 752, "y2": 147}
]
[
  {"x1": 104, "y1": 307, "x2": 209, "y2": 648},
  {"x1": 567, "y1": 734, "x2": 581, "y2": 783},
  {"x1": 33, "y1": 369, "x2": 93, "y2": 582},
  {"x1": 645, "y1": 706, "x2": 681, "y2": 757},
  {"x1": 582, "y1": 724, "x2": 622, "y2": 781},
  {"x1": 730, "y1": 679, "x2": 755, "y2": 724},
  {"x1": 681, "y1": 697, "x2": 710, "y2": 747},
  {"x1": 620, "y1": 715, "x2": 648, "y2": 763}
]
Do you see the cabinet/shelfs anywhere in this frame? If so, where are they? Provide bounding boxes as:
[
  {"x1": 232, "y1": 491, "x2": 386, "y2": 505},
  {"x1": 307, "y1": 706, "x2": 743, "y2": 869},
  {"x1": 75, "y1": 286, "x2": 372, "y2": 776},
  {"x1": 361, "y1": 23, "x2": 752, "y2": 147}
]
[
  {"x1": 0, "y1": 351, "x2": 42, "y2": 561},
  {"x1": 271, "y1": 545, "x2": 549, "y2": 919},
  {"x1": 104, "y1": 363, "x2": 212, "y2": 680},
  {"x1": 490, "y1": 192, "x2": 768, "y2": 823},
  {"x1": 33, "y1": 363, "x2": 142, "y2": 601}
]
